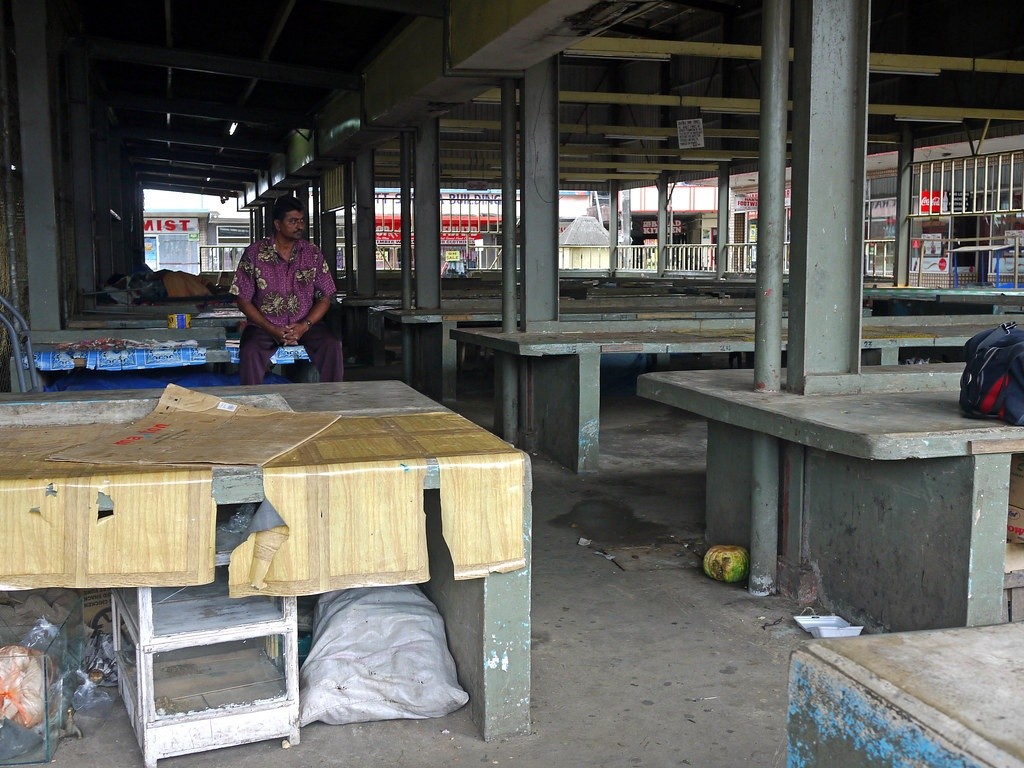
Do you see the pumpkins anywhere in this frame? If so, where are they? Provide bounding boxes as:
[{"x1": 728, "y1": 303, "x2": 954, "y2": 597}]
[{"x1": 703, "y1": 545, "x2": 751, "y2": 584}]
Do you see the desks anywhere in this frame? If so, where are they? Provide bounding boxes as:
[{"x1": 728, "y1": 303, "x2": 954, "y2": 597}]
[{"x1": 0, "y1": 270, "x2": 1024, "y2": 768}]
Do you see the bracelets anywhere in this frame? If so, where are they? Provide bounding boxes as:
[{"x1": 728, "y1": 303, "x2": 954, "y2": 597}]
[{"x1": 306, "y1": 320, "x2": 312, "y2": 326}]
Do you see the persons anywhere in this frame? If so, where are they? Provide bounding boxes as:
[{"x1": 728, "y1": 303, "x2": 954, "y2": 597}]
[
  {"x1": 107, "y1": 265, "x2": 223, "y2": 295},
  {"x1": 228, "y1": 194, "x2": 345, "y2": 385}
]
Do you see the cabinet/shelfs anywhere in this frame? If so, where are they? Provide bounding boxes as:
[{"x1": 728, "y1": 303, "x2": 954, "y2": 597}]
[{"x1": 106, "y1": 551, "x2": 300, "y2": 768}]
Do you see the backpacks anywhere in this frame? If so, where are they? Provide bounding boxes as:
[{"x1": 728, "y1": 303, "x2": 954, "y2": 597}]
[{"x1": 959, "y1": 321, "x2": 1023, "y2": 427}]
